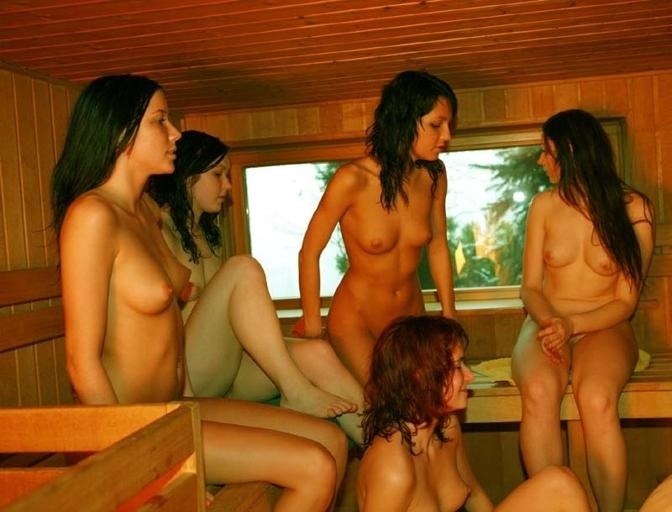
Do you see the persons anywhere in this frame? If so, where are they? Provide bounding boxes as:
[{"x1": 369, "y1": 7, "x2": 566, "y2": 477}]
[
  {"x1": 36, "y1": 72, "x2": 351, "y2": 510},
  {"x1": 147, "y1": 130, "x2": 373, "y2": 449},
  {"x1": 297, "y1": 70, "x2": 457, "y2": 391},
  {"x1": 353, "y1": 316, "x2": 591, "y2": 511},
  {"x1": 511, "y1": 109, "x2": 654, "y2": 511}
]
